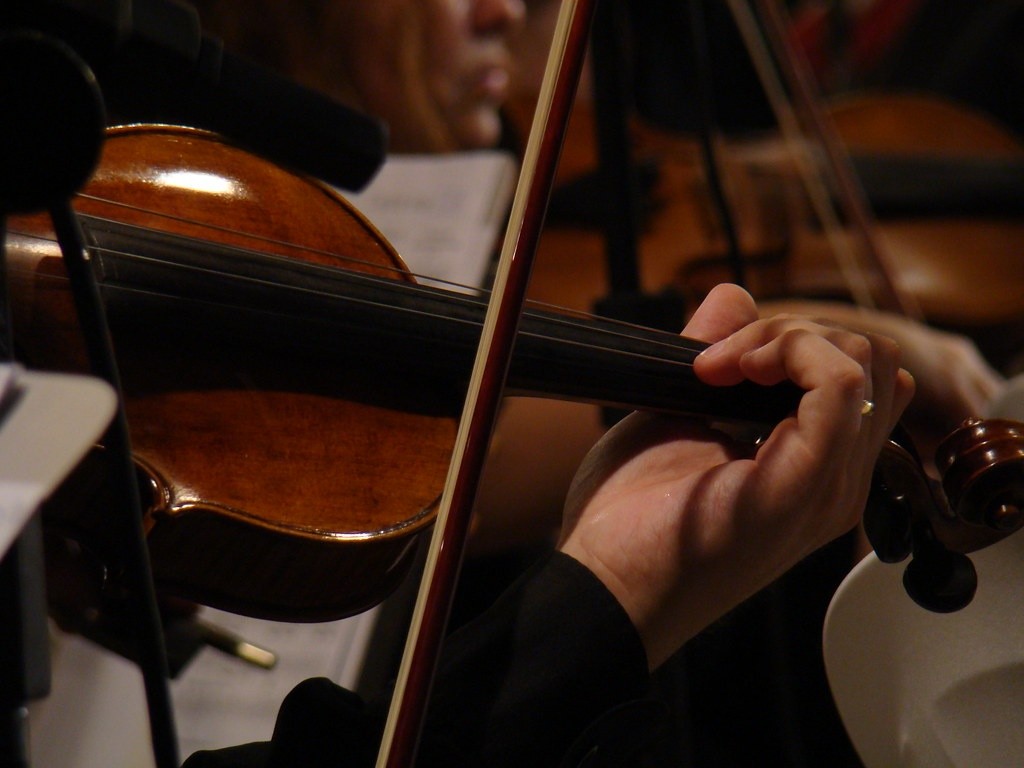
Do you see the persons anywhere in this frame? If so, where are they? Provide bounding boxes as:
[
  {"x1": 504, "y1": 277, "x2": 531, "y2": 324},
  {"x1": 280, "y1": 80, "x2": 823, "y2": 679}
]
[{"x1": 181, "y1": 0, "x2": 916, "y2": 767}]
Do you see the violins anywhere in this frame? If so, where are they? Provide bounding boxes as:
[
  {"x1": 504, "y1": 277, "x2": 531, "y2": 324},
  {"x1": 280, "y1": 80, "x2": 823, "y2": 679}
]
[{"x1": 0, "y1": 121, "x2": 1024, "y2": 624}]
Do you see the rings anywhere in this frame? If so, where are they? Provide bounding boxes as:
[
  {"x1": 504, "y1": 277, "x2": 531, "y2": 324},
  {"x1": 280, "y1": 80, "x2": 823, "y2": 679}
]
[{"x1": 861, "y1": 396, "x2": 875, "y2": 420}]
